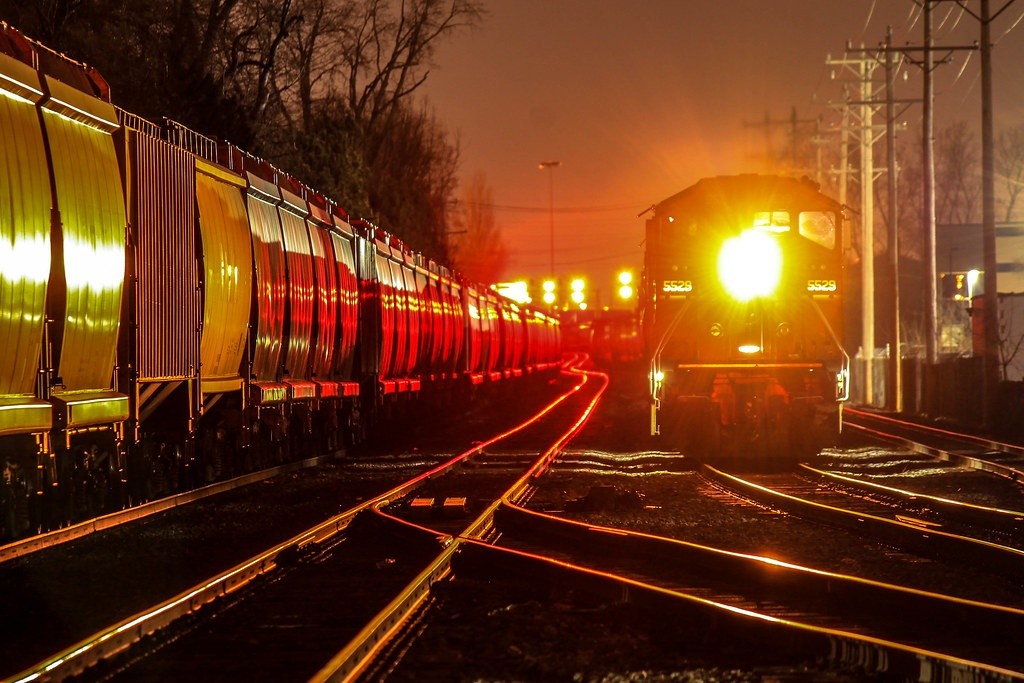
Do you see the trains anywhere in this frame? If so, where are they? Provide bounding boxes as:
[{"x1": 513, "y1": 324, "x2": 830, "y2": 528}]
[
  {"x1": 0, "y1": 14, "x2": 564, "y2": 517},
  {"x1": 552, "y1": 172, "x2": 855, "y2": 451}
]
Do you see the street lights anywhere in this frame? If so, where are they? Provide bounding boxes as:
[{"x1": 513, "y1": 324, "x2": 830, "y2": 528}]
[{"x1": 541, "y1": 159, "x2": 570, "y2": 300}]
